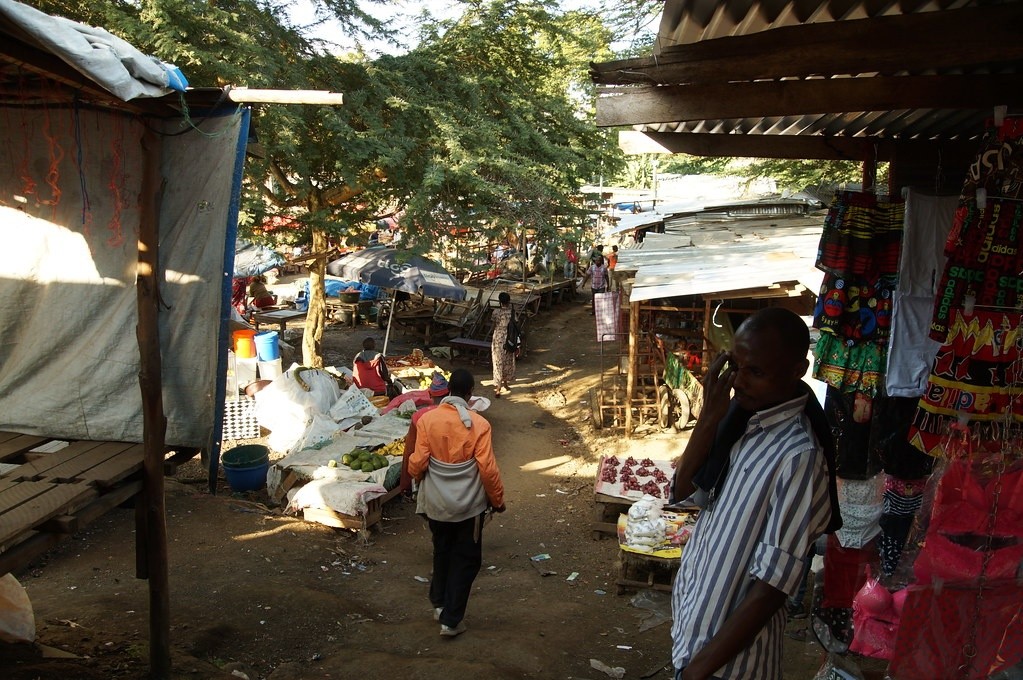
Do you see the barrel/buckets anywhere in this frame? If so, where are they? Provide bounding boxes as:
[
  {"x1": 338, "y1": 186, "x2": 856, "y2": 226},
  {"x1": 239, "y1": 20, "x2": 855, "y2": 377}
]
[
  {"x1": 254, "y1": 331, "x2": 279, "y2": 361},
  {"x1": 295, "y1": 298, "x2": 307, "y2": 311},
  {"x1": 232, "y1": 330, "x2": 257, "y2": 358}
]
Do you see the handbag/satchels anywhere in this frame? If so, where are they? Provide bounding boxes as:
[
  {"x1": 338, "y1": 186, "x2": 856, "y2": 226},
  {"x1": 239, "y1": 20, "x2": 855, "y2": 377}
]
[{"x1": 504, "y1": 310, "x2": 520, "y2": 352}]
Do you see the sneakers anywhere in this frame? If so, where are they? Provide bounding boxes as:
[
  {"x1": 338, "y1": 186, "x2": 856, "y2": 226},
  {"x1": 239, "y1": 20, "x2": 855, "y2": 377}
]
[
  {"x1": 440, "y1": 620, "x2": 467, "y2": 636},
  {"x1": 433, "y1": 608, "x2": 443, "y2": 620}
]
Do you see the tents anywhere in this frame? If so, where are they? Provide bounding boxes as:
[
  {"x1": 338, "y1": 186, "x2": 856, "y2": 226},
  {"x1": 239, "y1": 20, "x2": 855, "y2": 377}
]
[{"x1": 0, "y1": 0, "x2": 341, "y2": 451}]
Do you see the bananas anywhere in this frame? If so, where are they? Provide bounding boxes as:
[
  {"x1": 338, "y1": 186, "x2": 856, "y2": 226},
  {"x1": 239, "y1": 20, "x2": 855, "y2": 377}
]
[{"x1": 377, "y1": 436, "x2": 406, "y2": 456}]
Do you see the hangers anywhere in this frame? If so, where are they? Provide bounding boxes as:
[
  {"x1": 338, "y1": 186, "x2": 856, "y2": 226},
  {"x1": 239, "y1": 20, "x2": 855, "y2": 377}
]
[
  {"x1": 933, "y1": 544, "x2": 994, "y2": 594},
  {"x1": 832, "y1": 142, "x2": 907, "y2": 200},
  {"x1": 976, "y1": 182, "x2": 1023, "y2": 209},
  {"x1": 993, "y1": 106, "x2": 1023, "y2": 126},
  {"x1": 963, "y1": 292, "x2": 1022, "y2": 315},
  {"x1": 958, "y1": 412, "x2": 1023, "y2": 431},
  {"x1": 906, "y1": 148, "x2": 961, "y2": 196}
]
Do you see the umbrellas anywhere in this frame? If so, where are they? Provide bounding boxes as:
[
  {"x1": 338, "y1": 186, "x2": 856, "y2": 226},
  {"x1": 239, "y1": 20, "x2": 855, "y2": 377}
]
[{"x1": 325, "y1": 244, "x2": 467, "y2": 303}]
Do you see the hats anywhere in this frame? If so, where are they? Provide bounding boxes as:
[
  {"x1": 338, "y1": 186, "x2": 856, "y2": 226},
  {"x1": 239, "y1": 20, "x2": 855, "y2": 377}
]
[{"x1": 429, "y1": 370, "x2": 450, "y2": 396}]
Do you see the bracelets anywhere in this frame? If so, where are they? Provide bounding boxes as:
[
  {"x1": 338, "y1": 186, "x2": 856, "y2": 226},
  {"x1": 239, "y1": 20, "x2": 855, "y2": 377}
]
[{"x1": 675, "y1": 665, "x2": 688, "y2": 680}]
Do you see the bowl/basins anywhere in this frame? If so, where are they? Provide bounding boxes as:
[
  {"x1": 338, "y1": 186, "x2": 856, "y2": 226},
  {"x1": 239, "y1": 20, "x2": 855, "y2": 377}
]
[
  {"x1": 337, "y1": 290, "x2": 362, "y2": 303},
  {"x1": 244, "y1": 380, "x2": 273, "y2": 397},
  {"x1": 221, "y1": 445, "x2": 271, "y2": 491}
]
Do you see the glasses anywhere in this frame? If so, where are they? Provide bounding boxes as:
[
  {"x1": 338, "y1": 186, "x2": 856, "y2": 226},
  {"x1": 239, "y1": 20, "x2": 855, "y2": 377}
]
[{"x1": 728, "y1": 358, "x2": 772, "y2": 377}]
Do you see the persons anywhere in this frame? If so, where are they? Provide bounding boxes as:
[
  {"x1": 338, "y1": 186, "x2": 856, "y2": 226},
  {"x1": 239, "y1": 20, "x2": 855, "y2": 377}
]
[
  {"x1": 490, "y1": 290, "x2": 521, "y2": 398},
  {"x1": 400, "y1": 370, "x2": 452, "y2": 496},
  {"x1": 248, "y1": 275, "x2": 275, "y2": 308},
  {"x1": 666, "y1": 305, "x2": 843, "y2": 680},
  {"x1": 675, "y1": 335, "x2": 704, "y2": 374},
  {"x1": 406, "y1": 365, "x2": 506, "y2": 636},
  {"x1": 580, "y1": 244, "x2": 619, "y2": 317},
  {"x1": 352, "y1": 337, "x2": 395, "y2": 396}
]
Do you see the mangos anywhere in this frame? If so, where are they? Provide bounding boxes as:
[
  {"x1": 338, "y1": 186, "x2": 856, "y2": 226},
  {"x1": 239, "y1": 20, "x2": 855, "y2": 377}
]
[{"x1": 342, "y1": 448, "x2": 388, "y2": 472}]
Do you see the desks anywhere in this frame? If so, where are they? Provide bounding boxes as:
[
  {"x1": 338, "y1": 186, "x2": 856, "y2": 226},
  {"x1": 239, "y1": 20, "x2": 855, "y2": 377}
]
[
  {"x1": 615, "y1": 516, "x2": 681, "y2": 595},
  {"x1": 593, "y1": 454, "x2": 675, "y2": 540},
  {"x1": 325, "y1": 297, "x2": 374, "y2": 328},
  {"x1": 271, "y1": 356, "x2": 454, "y2": 537},
  {"x1": 254, "y1": 308, "x2": 308, "y2": 346},
  {"x1": 448, "y1": 337, "x2": 491, "y2": 367}
]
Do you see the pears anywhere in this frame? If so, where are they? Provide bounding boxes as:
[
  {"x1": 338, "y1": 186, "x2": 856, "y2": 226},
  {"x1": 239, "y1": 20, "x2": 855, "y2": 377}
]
[{"x1": 418, "y1": 371, "x2": 453, "y2": 389}]
